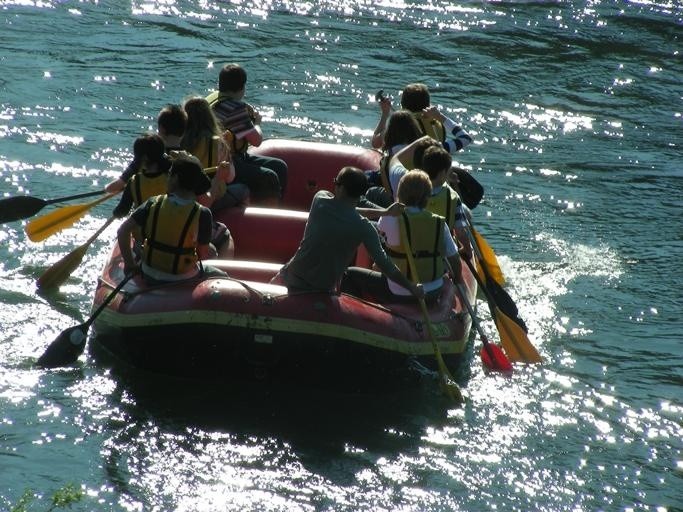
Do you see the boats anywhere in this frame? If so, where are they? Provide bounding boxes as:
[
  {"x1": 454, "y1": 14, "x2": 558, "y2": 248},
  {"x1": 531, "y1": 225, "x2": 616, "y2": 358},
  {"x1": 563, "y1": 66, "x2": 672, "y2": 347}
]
[{"x1": 91, "y1": 136, "x2": 477, "y2": 385}]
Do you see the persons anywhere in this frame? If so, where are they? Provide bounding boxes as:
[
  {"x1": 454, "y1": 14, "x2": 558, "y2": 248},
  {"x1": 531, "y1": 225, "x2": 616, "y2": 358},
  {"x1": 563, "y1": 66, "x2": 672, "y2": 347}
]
[
  {"x1": 203, "y1": 63, "x2": 289, "y2": 208},
  {"x1": 116, "y1": 159, "x2": 230, "y2": 287},
  {"x1": 268, "y1": 165, "x2": 426, "y2": 301},
  {"x1": 112, "y1": 98, "x2": 252, "y2": 260},
  {"x1": 338, "y1": 84, "x2": 474, "y2": 308}
]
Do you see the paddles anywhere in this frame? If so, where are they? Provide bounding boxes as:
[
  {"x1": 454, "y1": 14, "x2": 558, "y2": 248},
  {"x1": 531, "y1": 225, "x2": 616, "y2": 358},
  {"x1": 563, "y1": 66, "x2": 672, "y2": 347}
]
[
  {"x1": 37, "y1": 216, "x2": 119, "y2": 289},
  {"x1": 431, "y1": 119, "x2": 542, "y2": 370},
  {"x1": 35, "y1": 269, "x2": 140, "y2": 367},
  {"x1": 396, "y1": 213, "x2": 466, "y2": 404},
  {"x1": 376, "y1": 89, "x2": 483, "y2": 210},
  {"x1": 0, "y1": 165, "x2": 219, "y2": 242}
]
